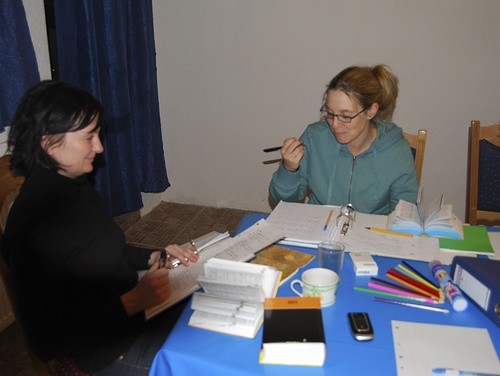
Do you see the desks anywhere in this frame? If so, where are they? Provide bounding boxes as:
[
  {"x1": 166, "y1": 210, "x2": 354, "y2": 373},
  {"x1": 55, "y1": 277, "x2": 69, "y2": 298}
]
[{"x1": 148, "y1": 208, "x2": 500, "y2": 375}]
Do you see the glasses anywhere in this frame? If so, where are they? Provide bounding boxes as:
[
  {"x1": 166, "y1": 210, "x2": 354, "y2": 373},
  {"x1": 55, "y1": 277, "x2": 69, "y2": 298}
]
[{"x1": 319, "y1": 101, "x2": 369, "y2": 124}]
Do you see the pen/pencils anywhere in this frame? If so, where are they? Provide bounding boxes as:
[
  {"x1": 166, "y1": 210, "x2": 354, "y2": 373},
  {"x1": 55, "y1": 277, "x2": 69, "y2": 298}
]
[
  {"x1": 160, "y1": 248, "x2": 167, "y2": 269},
  {"x1": 365, "y1": 227, "x2": 413, "y2": 238},
  {"x1": 432, "y1": 367, "x2": 500, "y2": 376},
  {"x1": 353, "y1": 259, "x2": 450, "y2": 314},
  {"x1": 323, "y1": 209, "x2": 333, "y2": 231},
  {"x1": 263, "y1": 142, "x2": 305, "y2": 153}
]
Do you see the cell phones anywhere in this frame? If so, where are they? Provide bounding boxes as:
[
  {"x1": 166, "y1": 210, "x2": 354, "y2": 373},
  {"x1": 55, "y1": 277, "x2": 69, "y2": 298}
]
[{"x1": 347, "y1": 312, "x2": 374, "y2": 341}]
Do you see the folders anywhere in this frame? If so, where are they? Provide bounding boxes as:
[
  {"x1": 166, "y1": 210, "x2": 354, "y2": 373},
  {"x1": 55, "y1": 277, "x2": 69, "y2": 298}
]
[{"x1": 448, "y1": 255, "x2": 500, "y2": 328}]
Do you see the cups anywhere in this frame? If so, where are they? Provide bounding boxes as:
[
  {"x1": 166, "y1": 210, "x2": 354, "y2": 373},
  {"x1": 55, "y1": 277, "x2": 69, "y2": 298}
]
[
  {"x1": 290, "y1": 268, "x2": 339, "y2": 309},
  {"x1": 318, "y1": 241, "x2": 345, "y2": 276}
]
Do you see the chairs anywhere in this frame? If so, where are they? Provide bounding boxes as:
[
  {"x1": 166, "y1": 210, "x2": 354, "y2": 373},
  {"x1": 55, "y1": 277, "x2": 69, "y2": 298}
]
[
  {"x1": 395, "y1": 128, "x2": 428, "y2": 186},
  {"x1": 0, "y1": 156, "x2": 88, "y2": 376},
  {"x1": 463, "y1": 121, "x2": 500, "y2": 225}
]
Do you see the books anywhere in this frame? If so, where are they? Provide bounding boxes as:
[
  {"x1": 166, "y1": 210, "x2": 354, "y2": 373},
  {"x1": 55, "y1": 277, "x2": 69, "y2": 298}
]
[
  {"x1": 265, "y1": 200, "x2": 457, "y2": 266},
  {"x1": 439, "y1": 225, "x2": 496, "y2": 256},
  {"x1": 137, "y1": 217, "x2": 288, "y2": 320},
  {"x1": 387, "y1": 186, "x2": 465, "y2": 241},
  {"x1": 259, "y1": 296, "x2": 326, "y2": 367},
  {"x1": 246, "y1": 244, "x2": 316, "y2": 288},
  {"x1": 188, "y1": 257, "x2": 283, "y2": 339}
]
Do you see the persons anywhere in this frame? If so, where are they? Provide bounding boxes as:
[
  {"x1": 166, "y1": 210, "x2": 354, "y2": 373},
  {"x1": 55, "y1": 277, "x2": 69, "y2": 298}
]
[
  {"x1": 0, "y1": 81, "x2": 200, "y2": 375},
  {"x1": 269, "y1": 65, "x2": 418, "y2": 215}
]
